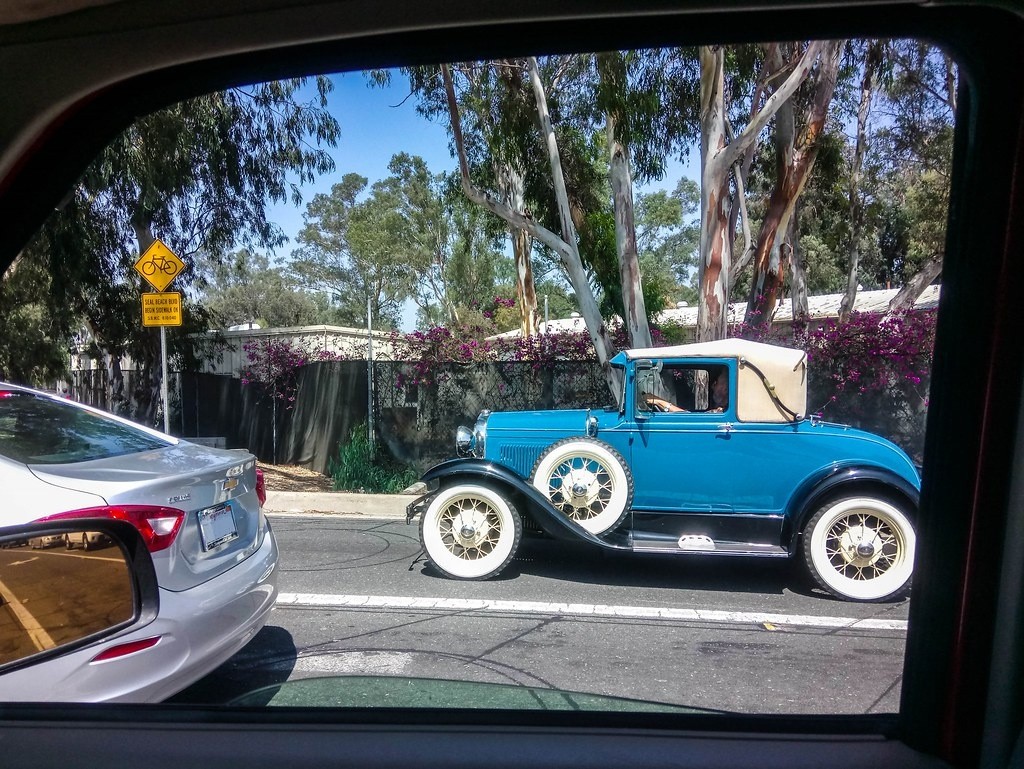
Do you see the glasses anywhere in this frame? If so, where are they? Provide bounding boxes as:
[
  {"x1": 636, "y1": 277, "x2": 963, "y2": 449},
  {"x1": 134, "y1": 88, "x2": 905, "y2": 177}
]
[{"x1": 714, "y1": 381, "x2": 726, "y2": 387}]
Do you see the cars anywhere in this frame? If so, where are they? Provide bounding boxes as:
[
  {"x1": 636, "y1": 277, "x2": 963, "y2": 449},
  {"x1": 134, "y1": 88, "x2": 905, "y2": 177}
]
[
  {"x1": 2, "y1": 381, "x2": 284, "y2": 704},
  {"x1": 404, "y1": 336, "x2": 921, "y2": 604}
]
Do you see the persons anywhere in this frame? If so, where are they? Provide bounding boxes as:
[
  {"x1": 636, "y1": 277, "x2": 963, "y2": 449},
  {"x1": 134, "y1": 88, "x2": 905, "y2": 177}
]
[{"x1": 641, "y1": 368, "x2": 730, "y2": 414}]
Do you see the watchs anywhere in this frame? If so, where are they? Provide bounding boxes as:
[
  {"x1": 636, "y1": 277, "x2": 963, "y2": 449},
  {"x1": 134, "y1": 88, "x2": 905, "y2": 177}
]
[{"x1": 664, "y1": 403, "x2": 672, "y2": 411}]
